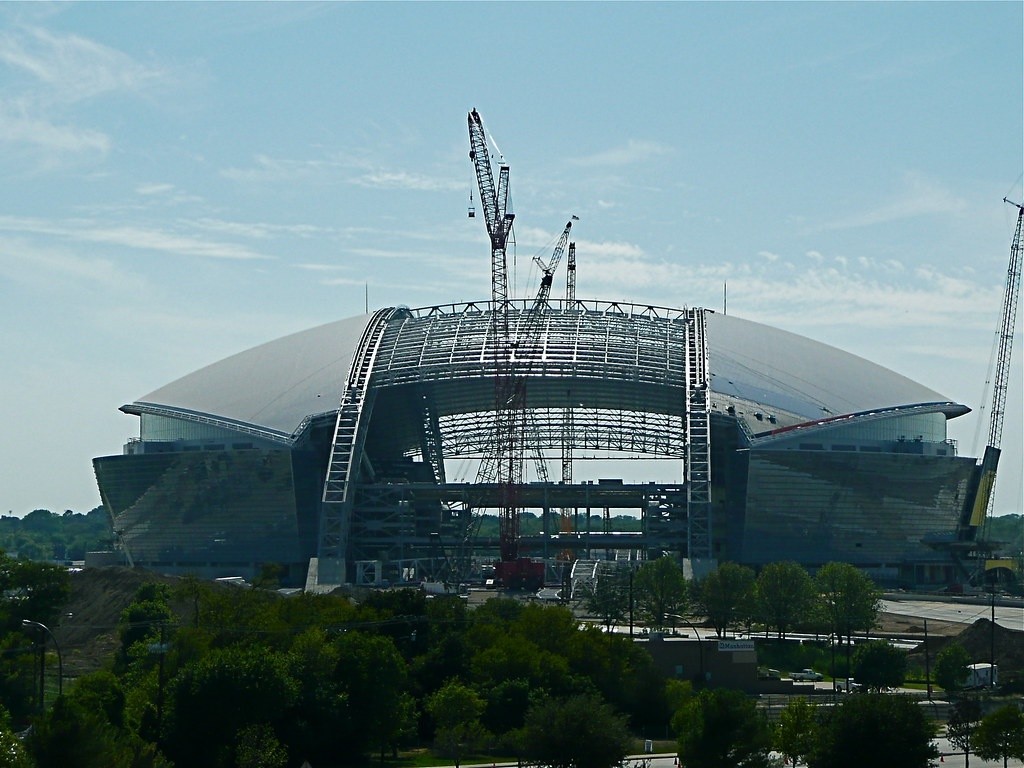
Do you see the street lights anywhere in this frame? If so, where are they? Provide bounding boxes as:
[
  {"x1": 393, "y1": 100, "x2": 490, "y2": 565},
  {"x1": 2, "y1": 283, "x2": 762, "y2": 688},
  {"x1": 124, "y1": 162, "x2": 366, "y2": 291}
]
[
  {"x1": 20, "y1": 618, "x2": 65, "y2": 697},
  {"x1": 664, "y1": 611, "x2": 704, "y2": 688}
]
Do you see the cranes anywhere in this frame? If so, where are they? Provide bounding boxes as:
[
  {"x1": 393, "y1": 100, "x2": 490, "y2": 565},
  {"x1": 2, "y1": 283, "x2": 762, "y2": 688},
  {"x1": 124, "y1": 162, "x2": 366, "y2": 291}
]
[
  {"x1": 937, "y1": 193, "x2": 1024, "y2": 597},
  {"x1": 420, "y1": 213, "x2": 578, "y2": 593}
]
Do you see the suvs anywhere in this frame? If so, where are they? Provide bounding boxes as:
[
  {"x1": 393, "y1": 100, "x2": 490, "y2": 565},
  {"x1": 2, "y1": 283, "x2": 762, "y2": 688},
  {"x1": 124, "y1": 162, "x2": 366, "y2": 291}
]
[{"x1": 217, "y1": 576, "x2": 253, "y2": 590}]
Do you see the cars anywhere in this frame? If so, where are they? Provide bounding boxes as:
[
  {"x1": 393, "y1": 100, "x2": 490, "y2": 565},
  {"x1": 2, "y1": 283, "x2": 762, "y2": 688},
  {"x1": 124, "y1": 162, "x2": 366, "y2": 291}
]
[{"x1": 757, "y1": 666, "x2": 782, "y2": 678}]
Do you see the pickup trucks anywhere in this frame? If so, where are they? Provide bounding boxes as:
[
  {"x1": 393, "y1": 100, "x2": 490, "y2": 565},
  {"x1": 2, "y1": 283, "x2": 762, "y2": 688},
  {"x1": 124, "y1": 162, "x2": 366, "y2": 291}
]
[
  {"x1": 787, "y1": 669, "x2": 824, "y2": 683},
  {"x1": 836, "y1": 678, "x2": 862, "y2": 694}
]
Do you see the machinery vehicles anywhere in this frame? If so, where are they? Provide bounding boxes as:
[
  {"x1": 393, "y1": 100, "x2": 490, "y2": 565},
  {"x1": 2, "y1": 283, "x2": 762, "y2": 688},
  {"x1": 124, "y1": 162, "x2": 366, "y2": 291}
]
[{"x1": 462, "y1": 104, "x2": 549, "y2": 592}]
